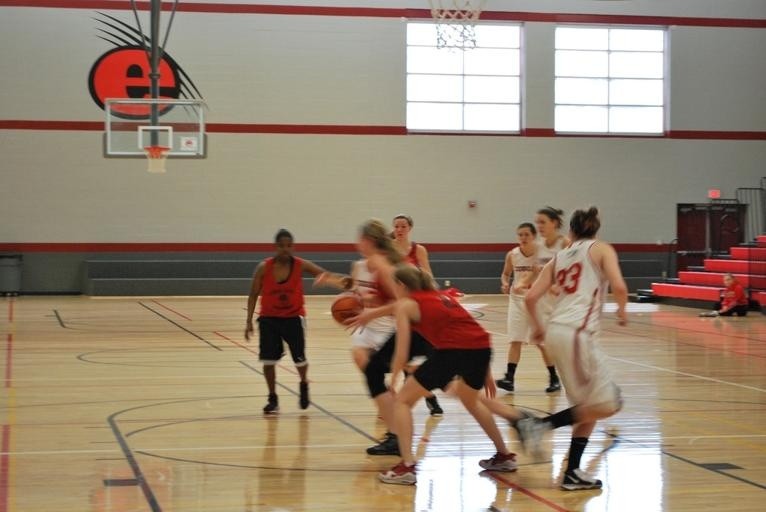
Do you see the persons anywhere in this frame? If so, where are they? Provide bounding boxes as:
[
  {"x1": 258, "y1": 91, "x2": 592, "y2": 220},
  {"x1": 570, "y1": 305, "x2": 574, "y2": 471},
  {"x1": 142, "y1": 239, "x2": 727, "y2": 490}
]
[
  {"x1": 343, "y1": 218, "x2": 534, "y2": 457},
  {"x1": 376, "y1": 262, "x2": 518, "y2": 486},
  {"x1": 512, "y1": 205, "x2": 632, "y2": 492},
  {"x1": 533, "y1": 206, "x2": 572, "y2": 318},
  {"x1": 389, "y1": 214, "x2": 432, "y2": 272},
  {"x1": 495, "y1": 222, "x2": 561, "y2": 393},
  {"x1": 699, "y1": 272, "x2": 749, "y2": 318},
  {"x1": 314, "y1": 257, "x2": 444, "y2": 418},
  {"x1": 244, "y1": 229, "x2": 326, "y2": 413}
]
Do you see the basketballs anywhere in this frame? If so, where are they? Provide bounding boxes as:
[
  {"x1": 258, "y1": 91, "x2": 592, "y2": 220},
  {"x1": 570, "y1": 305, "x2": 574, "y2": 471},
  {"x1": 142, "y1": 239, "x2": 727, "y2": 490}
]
[{"x1": 332, "y1": 293, "x2": 365, "y2": 326}]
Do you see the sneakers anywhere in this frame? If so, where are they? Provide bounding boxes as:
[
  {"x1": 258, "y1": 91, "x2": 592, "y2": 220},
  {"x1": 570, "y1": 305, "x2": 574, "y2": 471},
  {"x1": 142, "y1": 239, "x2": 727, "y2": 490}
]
[
  {"x1": 562, "y1": 468, "x2": 602, "y2": 491},
  {"x1": 496, "y1": 379, "x2": 514, "y2": 391},
  {"x1": 546, "y1": 383, "x2": 560, "y2": 392},
  {"x1": 367, "y1": 433, "x2": 400, "y2": 455},
  {"x1": 378, "y1": 461, "x2": 417, "y2": 485},
  {"x1": 426, "y1": 398, "x2": 443, "y2": 416},
  {"x1": 479, "y1": 451, "x2": 517, "y2": 472}
]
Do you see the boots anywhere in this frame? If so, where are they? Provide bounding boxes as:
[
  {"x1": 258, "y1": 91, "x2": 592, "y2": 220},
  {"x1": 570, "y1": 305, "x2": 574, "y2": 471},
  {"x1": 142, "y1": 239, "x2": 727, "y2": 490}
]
[
  {"x1": 299, "y1": 382, "x2": 309, "y2": 408},
  {"x1": 264, "y1": 394, "x2": 278, "y2": 412}
]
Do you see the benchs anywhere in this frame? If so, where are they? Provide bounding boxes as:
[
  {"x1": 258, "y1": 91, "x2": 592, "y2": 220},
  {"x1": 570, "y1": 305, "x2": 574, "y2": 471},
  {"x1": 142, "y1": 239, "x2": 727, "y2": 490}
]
[{"x1": 650, "y1": 233, "x2": 766, "y2": 310}]
